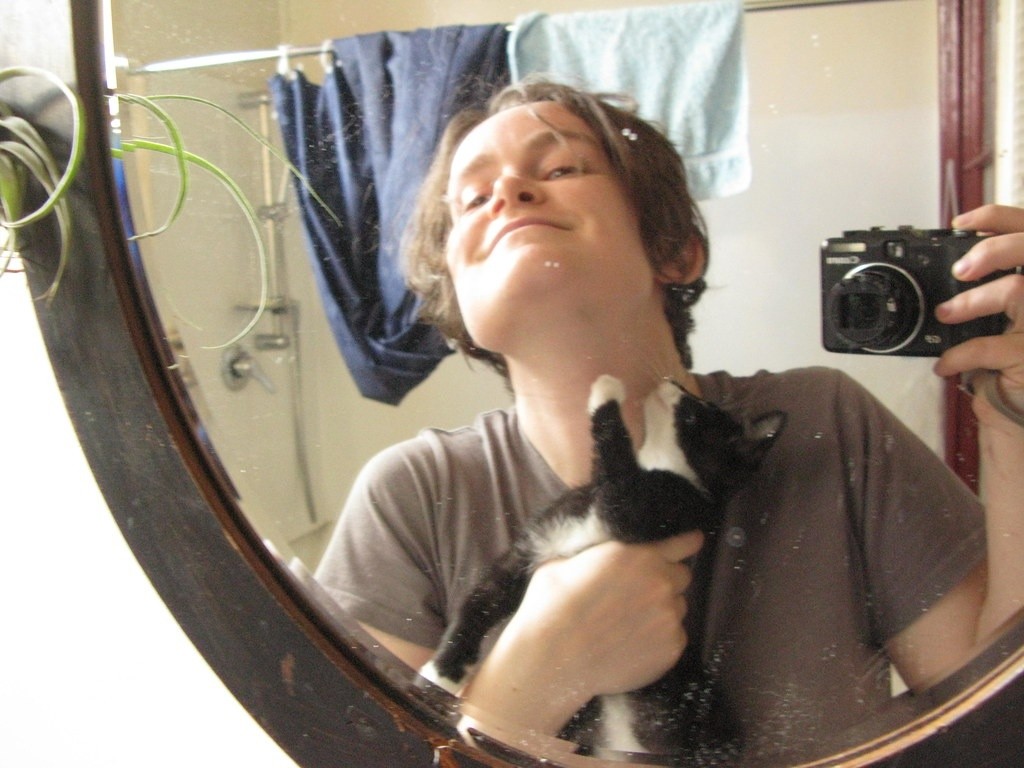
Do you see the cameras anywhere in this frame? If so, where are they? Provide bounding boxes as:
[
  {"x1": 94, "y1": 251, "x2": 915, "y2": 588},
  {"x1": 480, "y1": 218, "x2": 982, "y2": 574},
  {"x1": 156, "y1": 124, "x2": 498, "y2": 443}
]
[{"x1": 819, "y1": 225, "x2": 1008, "y2": 358}]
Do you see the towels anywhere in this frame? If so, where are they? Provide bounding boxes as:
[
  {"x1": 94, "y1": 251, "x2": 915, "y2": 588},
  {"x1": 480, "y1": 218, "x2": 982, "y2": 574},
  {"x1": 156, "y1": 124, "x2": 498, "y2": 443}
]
[{"x1": 506, "y1": 0, "x2": 751, "y2": 202}]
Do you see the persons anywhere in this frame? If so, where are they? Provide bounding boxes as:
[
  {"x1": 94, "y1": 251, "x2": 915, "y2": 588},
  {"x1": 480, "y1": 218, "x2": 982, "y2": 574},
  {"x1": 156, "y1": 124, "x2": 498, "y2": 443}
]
[{"x1": 314, "y1": 75, "x2": 1024, "y2": 755}]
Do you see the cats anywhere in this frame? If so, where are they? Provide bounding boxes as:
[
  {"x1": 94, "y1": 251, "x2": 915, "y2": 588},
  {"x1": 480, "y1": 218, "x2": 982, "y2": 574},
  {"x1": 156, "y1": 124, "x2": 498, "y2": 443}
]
[{"x1": 421, "y1": 371, "x2": 787, "y2": 768}]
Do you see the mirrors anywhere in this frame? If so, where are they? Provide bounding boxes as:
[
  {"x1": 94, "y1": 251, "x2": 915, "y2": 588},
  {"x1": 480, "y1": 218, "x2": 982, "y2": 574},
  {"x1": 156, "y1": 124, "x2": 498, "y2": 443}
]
[{"x1": 1, "y1": 0, "x2": 1023, "y2": 767}]
